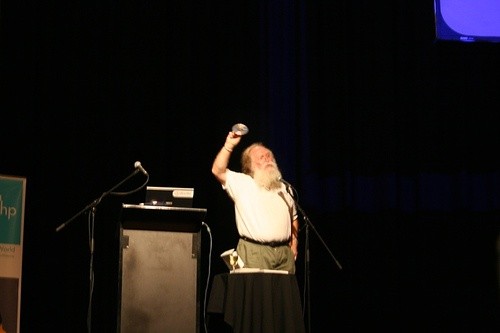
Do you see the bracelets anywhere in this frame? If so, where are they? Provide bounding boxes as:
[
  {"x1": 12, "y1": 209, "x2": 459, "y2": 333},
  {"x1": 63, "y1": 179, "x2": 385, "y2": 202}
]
[{"x1": 223, "y1": 146, "x2": 234, "y2": 152}]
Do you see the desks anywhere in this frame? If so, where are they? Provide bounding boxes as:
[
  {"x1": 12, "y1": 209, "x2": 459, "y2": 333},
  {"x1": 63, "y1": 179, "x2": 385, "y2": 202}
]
[{"x1": 207, "y1": 272, "x2": 305, "y2": 333}]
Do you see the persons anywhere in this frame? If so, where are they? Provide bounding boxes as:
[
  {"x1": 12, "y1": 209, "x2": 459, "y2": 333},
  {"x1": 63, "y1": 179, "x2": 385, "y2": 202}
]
[{"x1": 212, "y1": 130, "x2": 300, "y2": 275}]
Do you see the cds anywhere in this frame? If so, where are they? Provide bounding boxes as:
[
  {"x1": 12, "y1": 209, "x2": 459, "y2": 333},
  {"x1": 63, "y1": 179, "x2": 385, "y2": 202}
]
[{"x1": 232, "y1": 123, "x2": 249, "y2": 136}]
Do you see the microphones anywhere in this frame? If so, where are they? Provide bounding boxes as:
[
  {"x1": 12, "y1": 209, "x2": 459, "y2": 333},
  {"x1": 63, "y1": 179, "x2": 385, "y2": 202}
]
[
  {"x1": 134, "y1": 161, "x2": 148, "y2": 175},
  {"x1": 276, "y1": 176, "x2": 292, "y2": 187}
]
